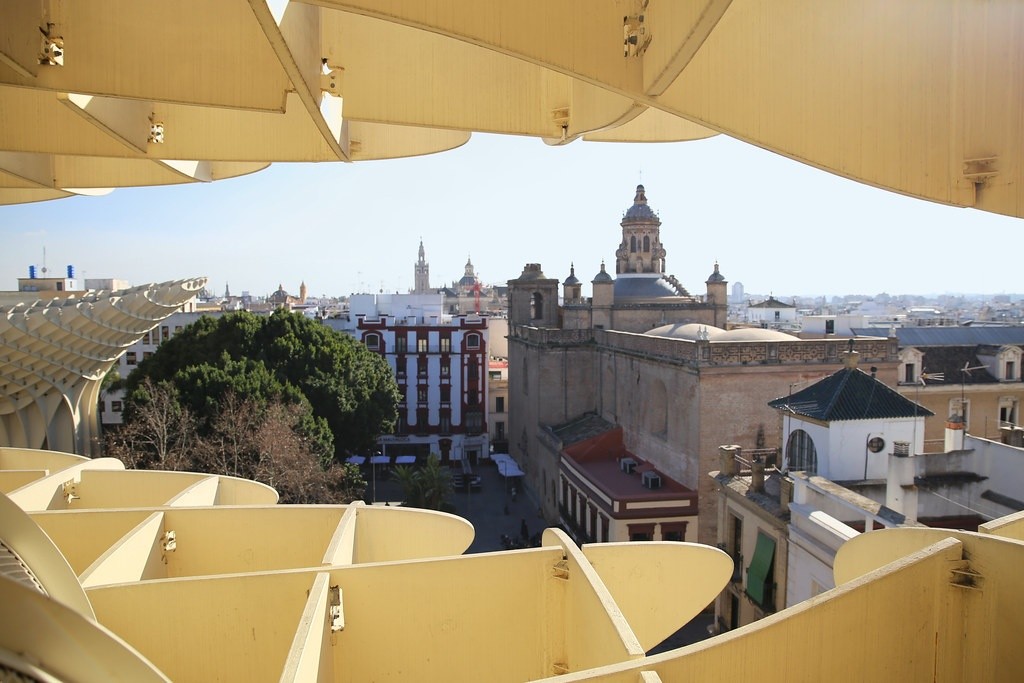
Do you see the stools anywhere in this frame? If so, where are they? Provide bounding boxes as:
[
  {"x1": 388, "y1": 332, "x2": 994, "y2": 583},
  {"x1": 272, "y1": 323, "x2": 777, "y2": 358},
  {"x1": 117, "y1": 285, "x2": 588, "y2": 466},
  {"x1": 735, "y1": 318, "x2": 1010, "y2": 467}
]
[
  {"x1": 621, "y1": 458, "x2": 638, "y2": 473},
  {"x1": 642, "y1": 471, "x2": 661, "y2": 488}
]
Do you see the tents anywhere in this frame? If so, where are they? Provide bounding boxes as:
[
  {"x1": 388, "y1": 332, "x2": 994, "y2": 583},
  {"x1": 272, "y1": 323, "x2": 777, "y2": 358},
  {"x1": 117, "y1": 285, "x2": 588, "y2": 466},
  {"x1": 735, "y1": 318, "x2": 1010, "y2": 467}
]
[
  {"x1": 491, "y1": 453, "x2": 526, "y2": 478},
  {"x1": 346, "y1": 455, "x2": 366, "y2": 465},
  {"x1": 370, "y1": 456, "x2": 390, "y2": 464},
  {"x1": 395, "y1": 455, "x2": 416, "y2": 463}
]
